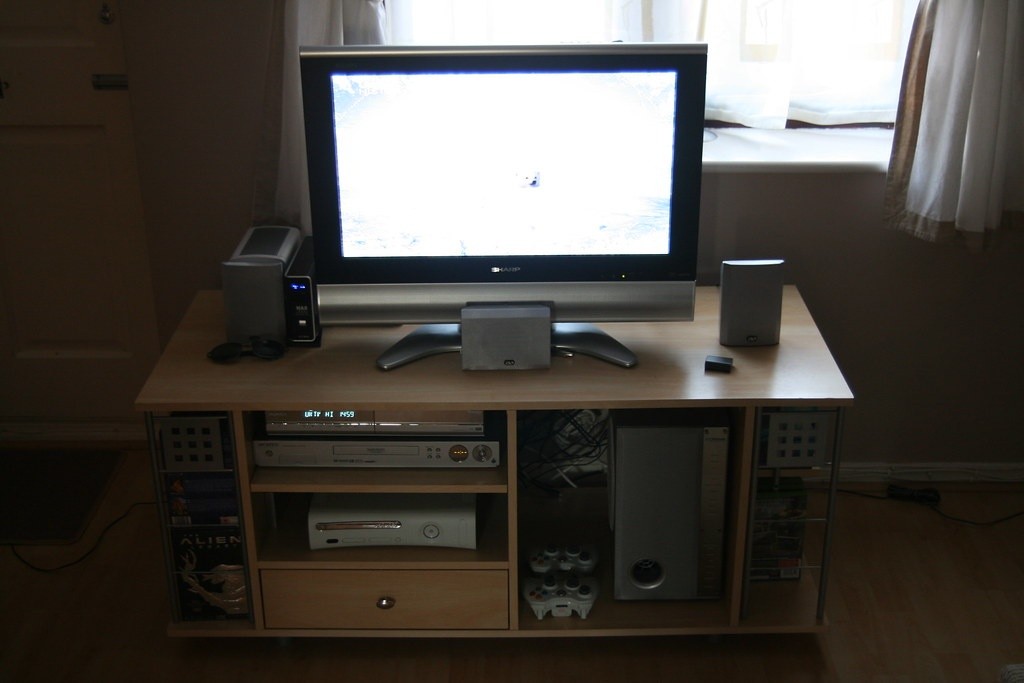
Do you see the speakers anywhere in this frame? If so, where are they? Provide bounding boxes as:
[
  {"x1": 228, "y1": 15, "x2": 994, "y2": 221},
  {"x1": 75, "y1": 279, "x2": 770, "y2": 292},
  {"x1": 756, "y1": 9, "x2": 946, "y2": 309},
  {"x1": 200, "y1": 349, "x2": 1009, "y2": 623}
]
[
  {"x1": 615, "y1": 425, "x2": 703, "y2": 602},
  {"x1": 461, "y1": 304, "x2": 551, "y2": 372},
  {"x1": 220, "y1": 226, "x2": 300, "y2": 349},
  {"x1": 719, "y1": 260, "x2": 784, "y2": 346}
]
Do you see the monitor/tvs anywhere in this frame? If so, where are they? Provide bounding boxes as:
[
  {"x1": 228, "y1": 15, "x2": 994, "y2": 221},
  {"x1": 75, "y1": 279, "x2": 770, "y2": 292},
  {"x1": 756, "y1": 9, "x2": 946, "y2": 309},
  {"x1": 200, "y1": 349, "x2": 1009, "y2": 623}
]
[{"x1": 299, "y1": 42, "x2": 709, "y2": 373}]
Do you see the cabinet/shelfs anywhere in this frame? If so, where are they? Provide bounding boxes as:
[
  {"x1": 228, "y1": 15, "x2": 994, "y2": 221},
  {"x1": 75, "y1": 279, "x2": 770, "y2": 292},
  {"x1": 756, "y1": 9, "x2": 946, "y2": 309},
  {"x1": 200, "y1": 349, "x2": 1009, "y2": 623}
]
[{"x1": 132, "y1": 283, "x2": 858, "y2": 638}]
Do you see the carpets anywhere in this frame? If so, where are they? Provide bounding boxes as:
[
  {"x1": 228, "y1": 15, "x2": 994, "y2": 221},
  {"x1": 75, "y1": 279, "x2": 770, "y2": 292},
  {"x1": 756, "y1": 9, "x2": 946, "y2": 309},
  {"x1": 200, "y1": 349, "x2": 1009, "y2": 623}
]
[{"x1": 0, "y1": 447, "x2": 128, "y2": 546}]
